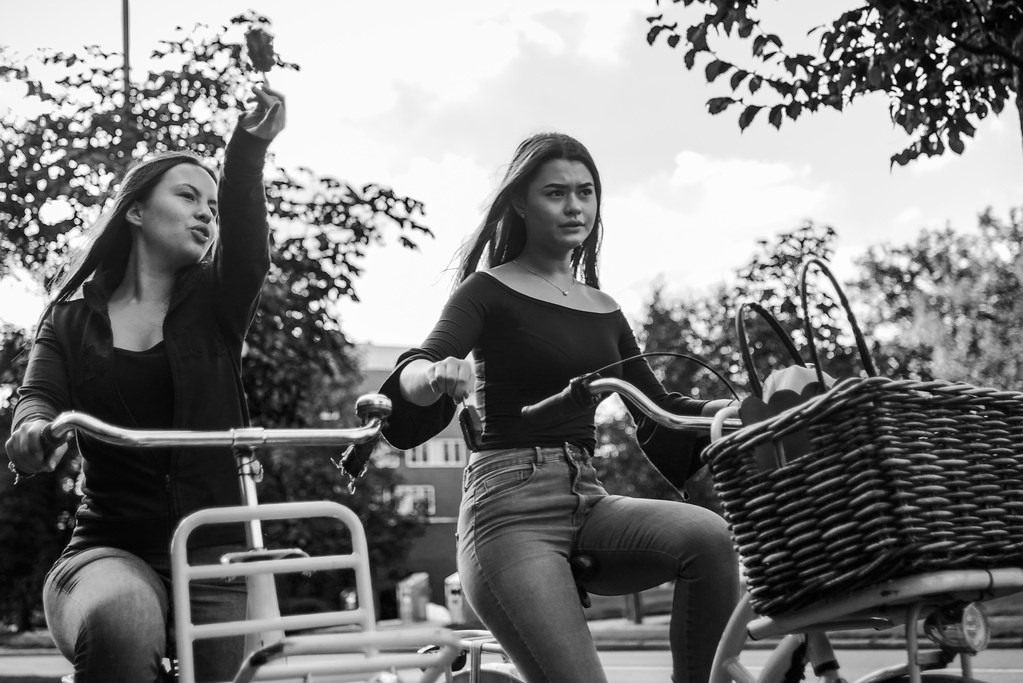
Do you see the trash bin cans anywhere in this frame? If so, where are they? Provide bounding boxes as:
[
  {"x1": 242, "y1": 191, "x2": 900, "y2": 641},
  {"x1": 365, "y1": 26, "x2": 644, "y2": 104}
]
[
  {"x1": 443, "y1": 571, "x2": 483, "y2": 624},
  {"x1": 397, "y1": 572, "x2": 429, "y2": 623}
]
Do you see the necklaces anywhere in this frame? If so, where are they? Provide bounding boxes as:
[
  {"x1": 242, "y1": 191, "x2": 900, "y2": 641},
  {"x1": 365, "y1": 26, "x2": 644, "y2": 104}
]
[{"x1": 513, "y1": 260, "x2": 576, "y2": 297}]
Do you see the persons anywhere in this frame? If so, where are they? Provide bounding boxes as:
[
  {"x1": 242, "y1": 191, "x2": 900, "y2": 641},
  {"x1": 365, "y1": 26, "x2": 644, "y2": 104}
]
[
  {"x1": 6, "y1": 77, "x2": 289, "y2": 681},
  {"x1": 371, "y1": 134, "x2": 743, "y2": 683}
]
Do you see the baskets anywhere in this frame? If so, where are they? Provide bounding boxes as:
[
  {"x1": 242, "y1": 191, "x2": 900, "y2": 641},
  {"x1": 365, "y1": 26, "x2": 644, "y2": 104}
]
[{"x1": 700, "y1": 376, "x2": 1022, "y2": 615}]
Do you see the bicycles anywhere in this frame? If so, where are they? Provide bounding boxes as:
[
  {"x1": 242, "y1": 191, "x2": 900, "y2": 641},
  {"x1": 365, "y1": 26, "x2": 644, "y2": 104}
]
[{"x1": 7, "y1": 252, "x2": 1022, "y2": 682}]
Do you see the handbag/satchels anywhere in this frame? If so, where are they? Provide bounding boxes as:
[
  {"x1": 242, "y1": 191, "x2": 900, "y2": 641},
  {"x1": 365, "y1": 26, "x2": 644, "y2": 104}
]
[{"x1": 731, "y1": 256, "x2": 877, "y2": 420}]
